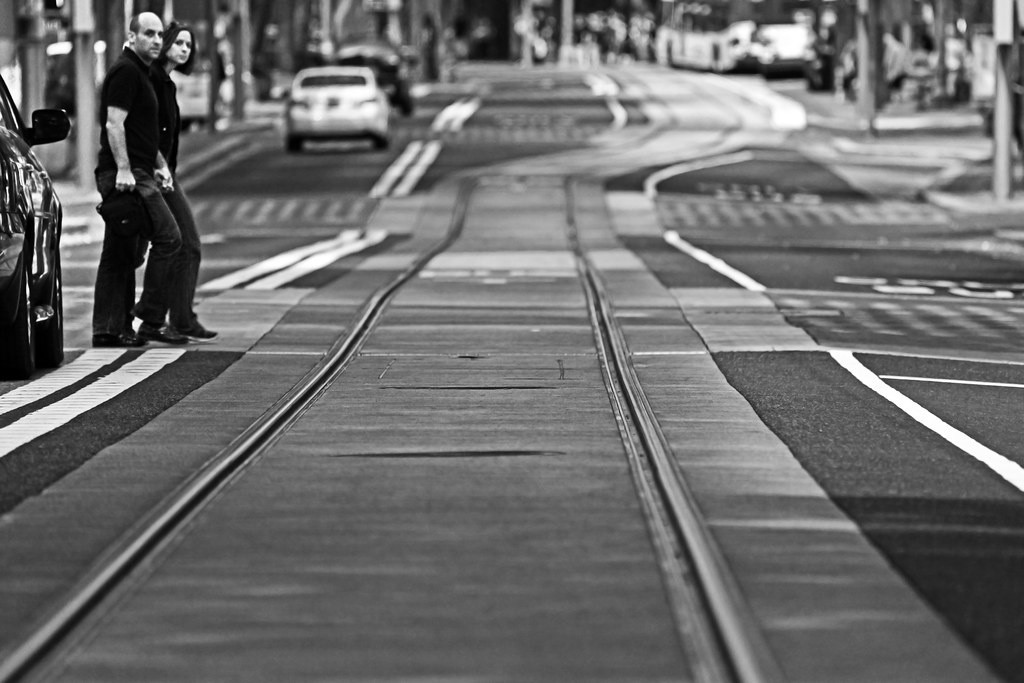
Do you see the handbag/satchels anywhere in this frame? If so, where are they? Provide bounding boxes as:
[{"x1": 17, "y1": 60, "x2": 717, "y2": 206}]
[{"x1": 97, "y1": 193, "x2": 147, "y2": 236}]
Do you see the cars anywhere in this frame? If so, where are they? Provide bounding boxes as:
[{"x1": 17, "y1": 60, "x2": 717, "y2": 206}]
[
  {"x1": 0, "y1": 74, "x2": 72, "y2": 380},
  {"x1": 340, "y1": 43, "x2": 413, "y2": 118},
  {"x1": 286, "y1": 64, "x2": 398, "y2": 155},
  {"x1": 757, "y1": 23, "x2": 818, "y2": 80}
]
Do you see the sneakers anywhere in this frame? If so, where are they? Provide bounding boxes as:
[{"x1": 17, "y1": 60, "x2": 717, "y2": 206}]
[{"x1": 174, "y1": 320, "x2": 218, "y2": 343}]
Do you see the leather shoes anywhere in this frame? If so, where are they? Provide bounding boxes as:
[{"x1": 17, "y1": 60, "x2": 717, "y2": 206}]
[
  {"x1": 139, "y1": 324, "x2": 188, "y2": 345},
  {"x1": 93, "y1": 331, "x2": 145, "y2": 349}
]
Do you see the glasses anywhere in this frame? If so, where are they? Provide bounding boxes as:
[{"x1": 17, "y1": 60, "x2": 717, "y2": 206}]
[{"x1": 166, "y1": 20, "x2": 190, "y2": 31}]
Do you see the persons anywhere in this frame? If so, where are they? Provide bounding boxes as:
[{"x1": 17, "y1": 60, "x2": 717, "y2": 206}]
[
  {"x1": 91, "y1": 12, "x2": 188, "y2": 348},
  {"x1": 134, "y1": 21, "x2": 219, "y2": 342}
]
